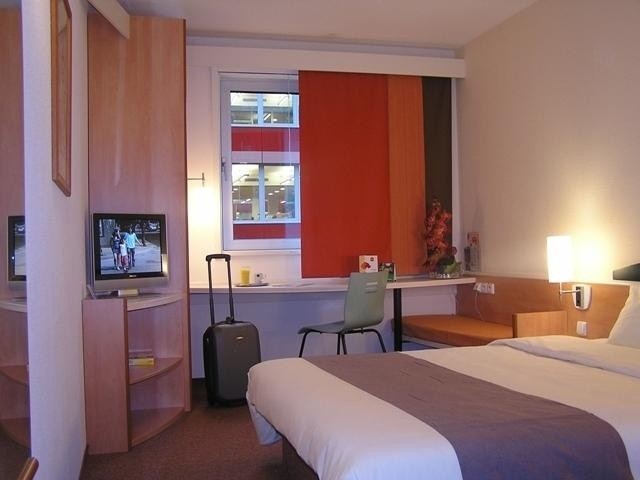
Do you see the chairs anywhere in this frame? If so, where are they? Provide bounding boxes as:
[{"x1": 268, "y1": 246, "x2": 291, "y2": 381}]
[{"x1": 296, "y1": 270, "x2": 389, "y2": 357}]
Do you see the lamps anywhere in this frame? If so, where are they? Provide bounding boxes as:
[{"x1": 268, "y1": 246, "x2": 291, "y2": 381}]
[{"x1": 545, "y1": 234, "x2": 592, "y2": 311}]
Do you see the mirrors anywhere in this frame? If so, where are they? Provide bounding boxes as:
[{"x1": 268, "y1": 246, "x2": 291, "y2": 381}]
[
  {"x1": 80, "y1": 292, "x2": 187, "y2": 456},
  {"x1": 0, "y1": 0, "x2": 38, "y2": 480}
]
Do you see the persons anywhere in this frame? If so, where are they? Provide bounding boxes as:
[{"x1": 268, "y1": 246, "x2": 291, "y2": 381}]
[
  {"x1": 124, "y1": 226, "x2": 143, "y2": 268},
  {"x1": 109, "y1": 228, "x2": 121, "y2": 271},
  {"x1": 119, "y1": 238, "x2": 130, "y2": 272}
]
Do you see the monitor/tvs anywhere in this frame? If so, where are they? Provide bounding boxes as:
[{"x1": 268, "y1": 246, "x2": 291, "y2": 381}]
[
  {"x1": 8, "y1": 216, "x2": 26, "y2": 303},
  {"x1": 91, "y1": 212, "x2": 168, "y2": 296}
]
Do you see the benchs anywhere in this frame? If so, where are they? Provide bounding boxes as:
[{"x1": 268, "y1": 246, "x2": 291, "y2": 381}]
[{"x1": 390, "y1": 274, "x2": 568, "y2": 347}]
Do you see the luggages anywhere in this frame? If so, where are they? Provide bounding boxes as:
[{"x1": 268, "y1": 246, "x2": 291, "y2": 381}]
[{"x1": 205, "y1": 254, "x2": 263, "y2": 409}]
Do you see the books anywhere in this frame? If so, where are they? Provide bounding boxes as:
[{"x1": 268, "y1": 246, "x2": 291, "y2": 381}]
[
  {"x1": 358, "y1": 254, "x2": 378, "y2": 273},
  {"x1": 129, "y1": 353, "x2": 154, "y2": 366}
]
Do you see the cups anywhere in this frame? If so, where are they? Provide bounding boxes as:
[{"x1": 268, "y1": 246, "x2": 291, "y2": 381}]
[
  {"x1": 240, "y1": 266, "x2": 250, "y2": 284},
  {"x1": 253, "y1": 272, "x2": 267, "y2": 284}
]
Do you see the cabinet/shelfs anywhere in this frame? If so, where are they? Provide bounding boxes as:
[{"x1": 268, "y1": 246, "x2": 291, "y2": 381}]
[{"x1": 0, "y1": 296, "x2": 30, "y2": 451}]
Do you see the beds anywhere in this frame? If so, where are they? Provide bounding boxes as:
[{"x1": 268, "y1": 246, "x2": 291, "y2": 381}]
[{"x1": 244, "y1": 284, "x2": 639, "y2": 480}]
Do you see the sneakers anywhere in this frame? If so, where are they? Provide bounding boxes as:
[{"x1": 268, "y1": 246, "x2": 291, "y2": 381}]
[{"x1": 113, "y1": 266, "x2": 129, "y2": 271}]
